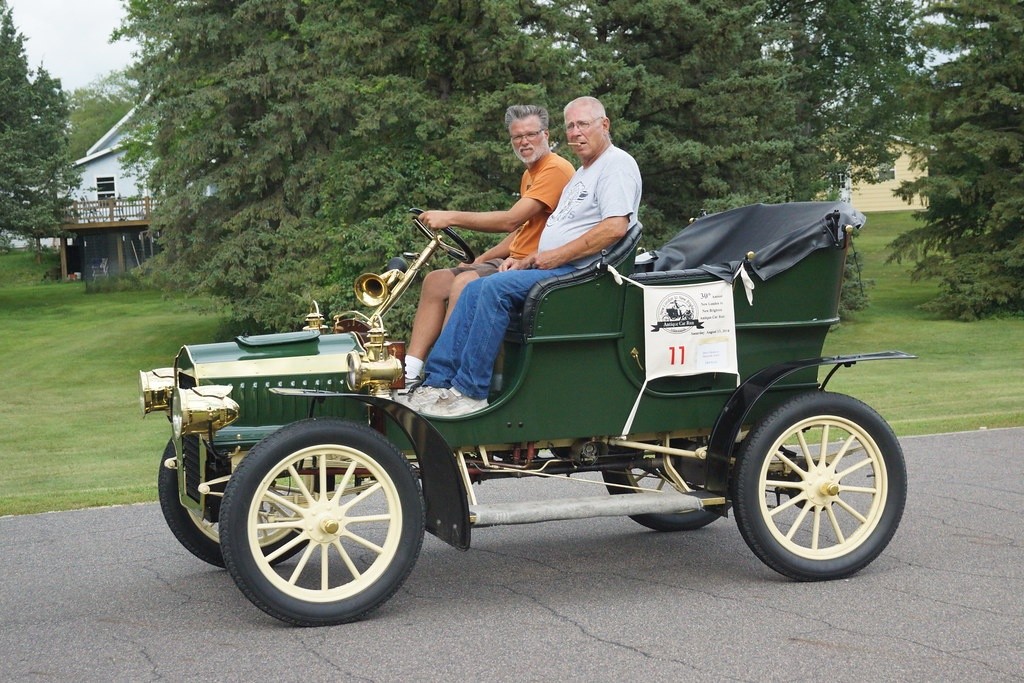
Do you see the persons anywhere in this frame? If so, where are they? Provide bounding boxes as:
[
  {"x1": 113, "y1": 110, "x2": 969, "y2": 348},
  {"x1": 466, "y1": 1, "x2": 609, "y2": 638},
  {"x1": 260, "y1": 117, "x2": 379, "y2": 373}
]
[{"x1": 392, "y1": 95, "x2": 643, "y2": 419}]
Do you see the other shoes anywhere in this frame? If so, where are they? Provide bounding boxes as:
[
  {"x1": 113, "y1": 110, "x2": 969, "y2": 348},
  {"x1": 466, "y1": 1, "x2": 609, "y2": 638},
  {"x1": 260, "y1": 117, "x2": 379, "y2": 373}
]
[{"x1": 394, "y1": 377, "x2": 424, "y2": 395}]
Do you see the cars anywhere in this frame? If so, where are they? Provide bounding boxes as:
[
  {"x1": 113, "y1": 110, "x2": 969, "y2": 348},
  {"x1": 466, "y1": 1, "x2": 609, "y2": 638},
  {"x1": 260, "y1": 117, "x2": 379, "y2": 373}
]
[{"x1": 139, "y1": 199, "x2": 917, "y2": 627}]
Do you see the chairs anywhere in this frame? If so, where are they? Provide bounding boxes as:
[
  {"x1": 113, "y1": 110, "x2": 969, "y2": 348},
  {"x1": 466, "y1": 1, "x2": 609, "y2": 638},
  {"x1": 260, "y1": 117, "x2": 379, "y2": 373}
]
[{"x1": 501, "y1": 220, "x2": 644, "y2": 343}]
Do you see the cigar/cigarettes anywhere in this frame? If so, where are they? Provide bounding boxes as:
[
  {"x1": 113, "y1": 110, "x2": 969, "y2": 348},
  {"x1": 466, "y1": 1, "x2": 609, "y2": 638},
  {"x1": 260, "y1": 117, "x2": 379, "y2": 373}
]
[{"x1": 568, "y1": 142, "x2": 581, "y2": 147}]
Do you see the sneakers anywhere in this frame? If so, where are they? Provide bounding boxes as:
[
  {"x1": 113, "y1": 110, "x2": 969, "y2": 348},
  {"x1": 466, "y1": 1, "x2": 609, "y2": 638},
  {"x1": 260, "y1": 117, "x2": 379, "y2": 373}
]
[
  {"x1": 404, "y1": 386, "x2": 448, "y2": 414},
  {"x1": 419, "y1": 386, "x2": 488, "y2": 414}
]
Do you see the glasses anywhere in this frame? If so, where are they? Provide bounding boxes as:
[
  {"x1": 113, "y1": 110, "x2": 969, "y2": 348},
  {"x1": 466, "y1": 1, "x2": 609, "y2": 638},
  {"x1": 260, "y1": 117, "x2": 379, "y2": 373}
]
[
  {"x1": 509, "y1": 128, "x2": 545, "y2": 143},
  {"x1": 562, "y1": 116, "x2": 603, "y2": 134}
]
